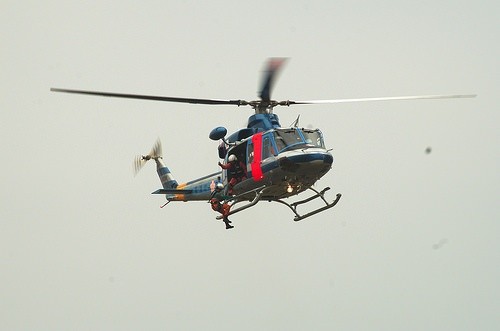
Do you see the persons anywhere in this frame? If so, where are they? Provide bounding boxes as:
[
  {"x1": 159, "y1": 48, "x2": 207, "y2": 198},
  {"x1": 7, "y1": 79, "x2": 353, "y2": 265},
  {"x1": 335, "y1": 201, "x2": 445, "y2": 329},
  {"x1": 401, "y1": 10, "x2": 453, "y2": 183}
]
[
  {"x1": 218, "y1": 154, "x2": 247, "y2": 197},
  {"x1": 269, "y1": 135, "x2": 287, "y2": 154},
  {"x1": 211, "y1": 183, "x2": 234, "y2": 229}
]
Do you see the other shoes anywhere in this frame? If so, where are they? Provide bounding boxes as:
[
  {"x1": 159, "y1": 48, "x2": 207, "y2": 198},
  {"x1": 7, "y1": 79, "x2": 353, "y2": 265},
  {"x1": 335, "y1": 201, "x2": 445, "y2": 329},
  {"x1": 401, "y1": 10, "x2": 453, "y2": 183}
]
[
  {"x1": 226, "y1": 225, "x2": 234, "y2": 229},
  {"x1": 223, "y1": 218, "x2": 232, "y2": 223}
]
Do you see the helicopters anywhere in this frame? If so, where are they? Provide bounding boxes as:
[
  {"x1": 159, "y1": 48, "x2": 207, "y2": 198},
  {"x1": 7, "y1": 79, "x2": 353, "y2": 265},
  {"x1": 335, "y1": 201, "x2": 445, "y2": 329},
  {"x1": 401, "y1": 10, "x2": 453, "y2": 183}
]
[{"x1": 50, "y1": 54, "x2": 480, "y2": 229}]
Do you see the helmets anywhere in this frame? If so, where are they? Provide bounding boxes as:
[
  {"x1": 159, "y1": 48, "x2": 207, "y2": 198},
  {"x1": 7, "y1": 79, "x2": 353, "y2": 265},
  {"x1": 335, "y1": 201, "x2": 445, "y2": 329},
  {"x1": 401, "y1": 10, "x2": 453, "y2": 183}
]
[
  {"x1": 217, "y1": 183, "x2": 224, "y2": 189},
  {"x1": 228, "y1": 154, "x2": 237, "y2": 162}
]
